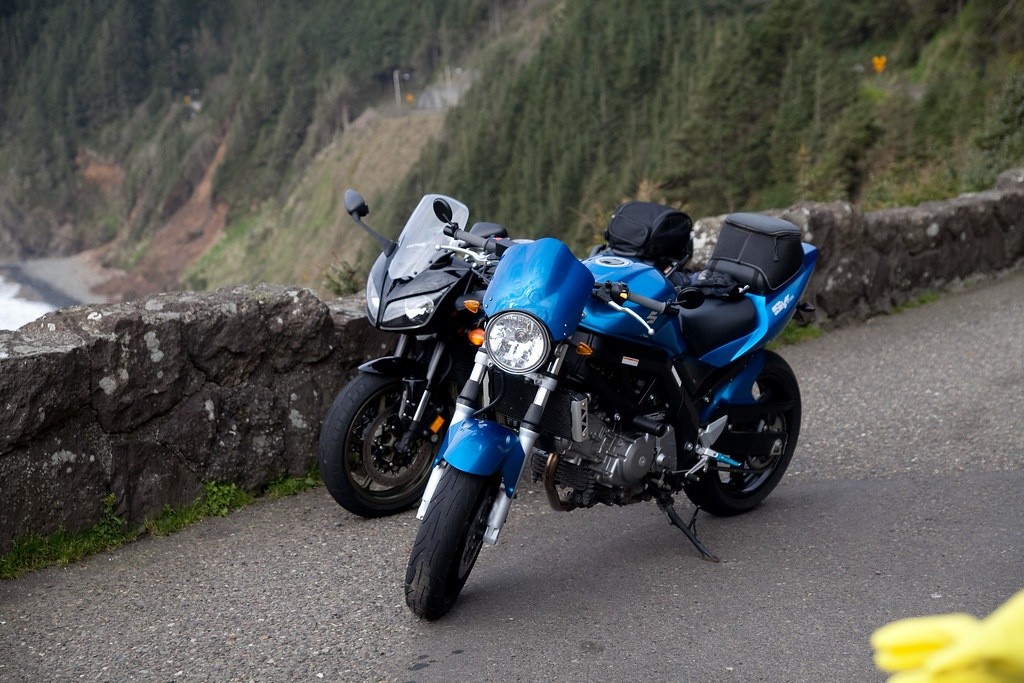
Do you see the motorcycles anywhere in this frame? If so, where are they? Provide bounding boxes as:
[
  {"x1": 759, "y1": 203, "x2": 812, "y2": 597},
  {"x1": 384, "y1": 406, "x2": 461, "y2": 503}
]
[
  {"x1": 315, "y1": 187, "x2": 695, "y2": 520},
  {"x1": 401, "y1": 196, "x2": 820, "y2": 623}
]
